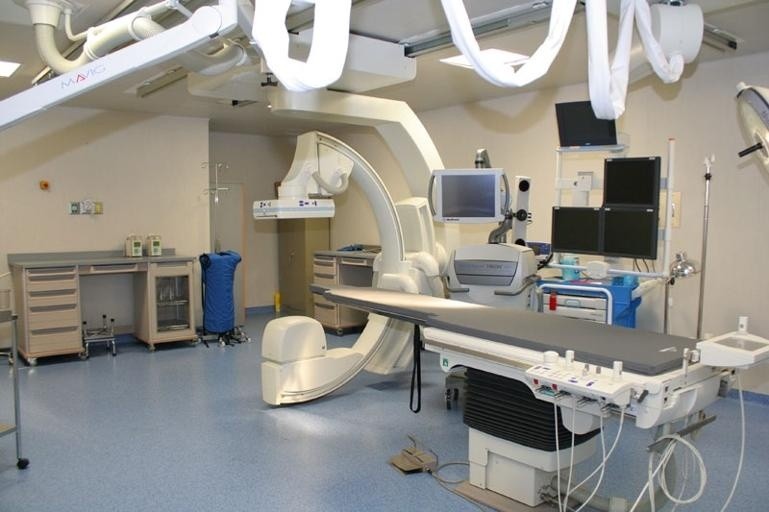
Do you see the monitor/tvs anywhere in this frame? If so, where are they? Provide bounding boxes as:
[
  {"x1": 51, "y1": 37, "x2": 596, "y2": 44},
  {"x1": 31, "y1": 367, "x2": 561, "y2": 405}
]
[
  {"x1": 604, "y1": 157, "x2": 660, "y2": 208},
  {"x1": 552, "y1": 206, "x2": 603, "y2": 255},
  {"x1": 601, "y1": 207, "x2": 658, "y2": 260},
  {"x1": 556, "y1": 101, "x2": 617, "y2": 146},
  {"x1": 436, "y1": 168, "x2": 501, "y2": 223}
]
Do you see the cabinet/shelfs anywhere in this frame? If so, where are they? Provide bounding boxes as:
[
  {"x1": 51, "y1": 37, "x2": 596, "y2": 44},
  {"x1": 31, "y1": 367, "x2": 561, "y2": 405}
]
[{"x1": 275, "y1": 181, "x2": 331, "y2": 311}]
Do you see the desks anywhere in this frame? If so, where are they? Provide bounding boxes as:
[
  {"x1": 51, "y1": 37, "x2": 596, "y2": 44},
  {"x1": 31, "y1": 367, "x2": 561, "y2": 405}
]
[
  {"x1": 312, "y1": 251, "x2": 377, "y2": 336},
  {"x1": 8, "y1": 248, "x2": 198, "y2": 366}
]
